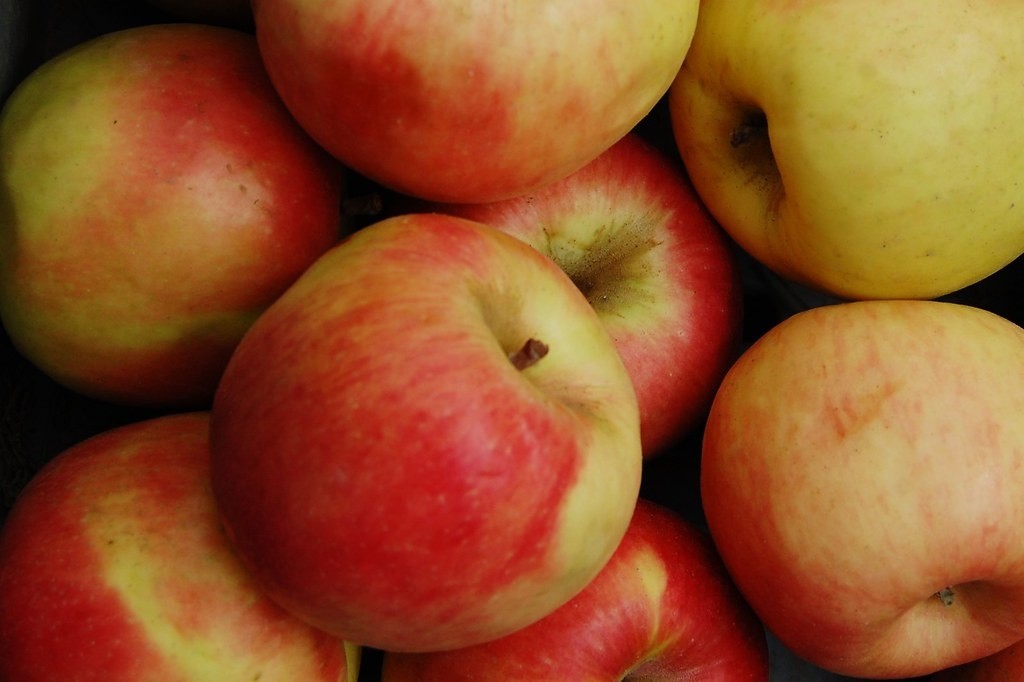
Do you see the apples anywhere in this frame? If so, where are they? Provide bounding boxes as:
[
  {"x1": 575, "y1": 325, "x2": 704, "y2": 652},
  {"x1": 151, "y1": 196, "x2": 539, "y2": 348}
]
[{"x1": 0, "y1": 0, "x2": 1024, "y2": 682}]
